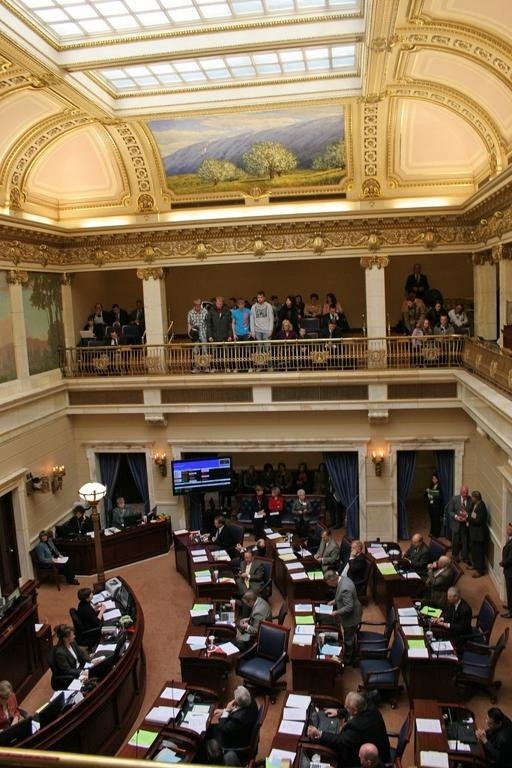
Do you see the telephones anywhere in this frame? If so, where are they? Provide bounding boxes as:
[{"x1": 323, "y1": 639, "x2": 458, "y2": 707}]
[{"x1": 162, "y1": 740, "x2": 178, "y2": 750}]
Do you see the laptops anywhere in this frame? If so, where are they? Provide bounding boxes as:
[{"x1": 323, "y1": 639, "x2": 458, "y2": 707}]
[{"x1": 80, "y1": 331, "x2": 96, "y2": 338}]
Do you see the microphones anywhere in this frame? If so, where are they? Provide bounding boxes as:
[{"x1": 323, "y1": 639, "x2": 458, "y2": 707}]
[
  {"x1": 424, "y1": 600, "x2": 433, "y2": 631},
  {"x1": 301, "y1": 545, "x2": 305, "y2": 559},
  {"x1": 172, "y1": 679, "x2": 176, "y2": 728},
  {"x1": 453, "y1": 708, "x2": 471, "y2": 751},
  {"x1": 391, "y1": 544, "x2": 395, "y2": 562},
  {"x1": 136, "y1": 730, "x2": 140, "y2": 758},
  {"x1": 435, "y1": 630, "x2": 445, "y2": 659},
  {"x1": 310, "y1": 563, "x2": 318, "y2": 584}
]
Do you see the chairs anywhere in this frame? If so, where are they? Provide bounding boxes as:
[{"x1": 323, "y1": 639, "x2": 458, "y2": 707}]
[
  {"x1": 239, "y1": 555, "x2": 275, "y2": 606},
  {"x1": 224, "y1": 696, "x2": 269, "y2": 768},
  {"x1": 353, "y1": 606, "x2": 407, "y2": 697},
  {"x1": 236, "y1": 620, "x2": 289, "y2": 705},
  {"x1": 385, "y1": 698, "x2": 416, "y2": 767},
  {"x1": 29, "y1": 543, "x2": 63, "y2": 591},
  {"x1": 412, "y1": 535, "x2": 465, "y2": 595},
  {"x1": 304, "y1": 532, "x2": 375, "y2": 601},
  {"x1": 452, "y1": 595, "x2": 511, "y2": 706}
]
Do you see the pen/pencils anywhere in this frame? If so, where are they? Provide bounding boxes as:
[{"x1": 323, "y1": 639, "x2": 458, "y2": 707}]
[{"x1": 298, "y1": 625, "x2": 300, "y2": 632}]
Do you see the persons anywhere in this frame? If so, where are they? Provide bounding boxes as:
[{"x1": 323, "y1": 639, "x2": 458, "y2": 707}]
[
  {"x1": 404, "y1": 264, "x2": 430, "y2": 297},
  {"x1": 195, "y1": 686, "x2": 259, "y2": 765},
  {"x1": 402, "y1": 292, "x2": 473, "y2": 367},
  {"x1": 499, "y1": 522, "x2": 511, "y2": 616},
  {"x1": 1, "y1": 679, "x2": 25, "y2": 732},
  {"x1": 476, "y1": 708, "x2": 510, "y2": 767},
  {"x1": 186, "y1": 292, "x2": 358, "y2": 373},
  {"x1": 209, "y1": 516, "x2": 274, "y2": 641},
  {"x1": 432, "y1": 587, "x2": 475, "y2": 647},
  {"x1": 78, "y1": 300, "x2": 148, "y2": 376},
  {"x1": 48, "y1": 589, "x2": 108, "y2": 692},
  {"x1": 35, "y1": 504, "x2": 92, "y2": 590},
  {"x1": 111, "y1": 497, "x2": 133, "y2": 528},
  {"x1": 405, "y1": 472, "x2": 490, "y2": 595},
  {"x1": 313, "y1": 528, "x2": 368, "y2": 636},
  {"x1": 241, "y1": 462, "x2": 344, "y2": 539},
  {"x1": 306, "y1": 689, "x2": 391, "y2": 768}
]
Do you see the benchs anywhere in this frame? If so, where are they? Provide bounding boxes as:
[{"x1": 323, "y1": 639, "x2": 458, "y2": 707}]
[
  {"x1": 231, "y1": 495, "x2": 326, "y2": 529},
  {"x1": 232, "y1": 469, "x2": 319, "y2": 495}
]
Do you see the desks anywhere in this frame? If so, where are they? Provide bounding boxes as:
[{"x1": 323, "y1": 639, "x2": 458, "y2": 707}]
[
  {"x1": 414, "y1": 698, "x2": 487, "y2": 767},
  {"x1": 362, "y1": 539, "x2": 421, "y2": 620},
  {"x1": 288, "y1": 597, "x2": 345, "y2": 695},
  {"x1": 117, "y1": 532, "x2": 238, "y2": 763},
  {"x1": 52, "y1": 513, "x2": 172, "y2": 577},
  {"x1": 262, "y1": 532, "x2": 328, "y2": 601},
  {"x1": 267, "y1": 689, "x2": 348, "y2": 767},
  {"x1": 390, "y1": 596, "x2": 458, "y2": 707}
]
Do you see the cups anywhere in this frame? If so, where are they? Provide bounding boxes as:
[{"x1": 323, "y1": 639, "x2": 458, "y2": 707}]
[
  {"x1": 214, "y1": 570, "x2": 219, "y2": 578},
  {"x1": 415, "y1": 601, "x2": 421, "y2": 609},
  {"x1": 209, "y1": 635, "x2": 216, "y2": 650},
  {"x1": 143, "y1": 516, "x2": 148, "y2": 523},
  {"x1": 425, "y1": 630, "x2": 433, "y2": 640},
  {"x1": 320, "y1": 633, "x2": 326, "y2": 644}
]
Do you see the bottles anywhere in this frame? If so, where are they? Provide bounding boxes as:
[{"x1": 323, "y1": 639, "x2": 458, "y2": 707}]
[
  {"x1": 188, "y1": 693, "x2": 194, "y2": 707},
  {"x1": 311, "y1": 753, "x2": 321, "y2": 767}
]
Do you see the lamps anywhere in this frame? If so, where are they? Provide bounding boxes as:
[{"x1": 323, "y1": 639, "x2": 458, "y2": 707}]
[
  {"x1": 52, "y1": 465, "x2": 66, "y2": 494},
  {"x1": 155, "y1": 453, "x2": 167, "y2": 477},
  {"x1": 372, "y1": 452, "x2": 384, "y2": 476}
]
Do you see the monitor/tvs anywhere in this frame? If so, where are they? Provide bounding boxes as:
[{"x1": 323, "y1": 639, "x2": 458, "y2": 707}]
[
  {"x1": 0, "y1": 716, "x2": 32, "y2": 747},
  {"x1": 120, "y1": 586, "x2": 129, "y2": 610},
  {"x1": 113, "y1": 633, "x2": 126, "y2": 657},
  {"x1": 124, "y1": 513, "x2": 142, "y2": 527},
  {"x1": 40, "y1": 692, "x2": 64, "y2": 730},
  {"x1": 55, "y1": 525, "x2": 79, "y2": 539},
  {"x1": 171, "y1": 456, "x2": 232, "y2": 496},
  {"x1": 90, "y1": 654, "x2": 116, "y2": 681}
]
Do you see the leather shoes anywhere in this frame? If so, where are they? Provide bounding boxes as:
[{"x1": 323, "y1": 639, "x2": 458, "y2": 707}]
[
  {"x1": 500, "y1": 614, "x2": 511, "y2": 618},
  {"x1": 454, "y1": 557, "x2": 487, "y2": 579},
  {"x1": 68, "y1": 580, "x2": 79, "y2": 585},
  {"x1": 503, "y1": 605, "x2": 511, "y2": 610}
]
